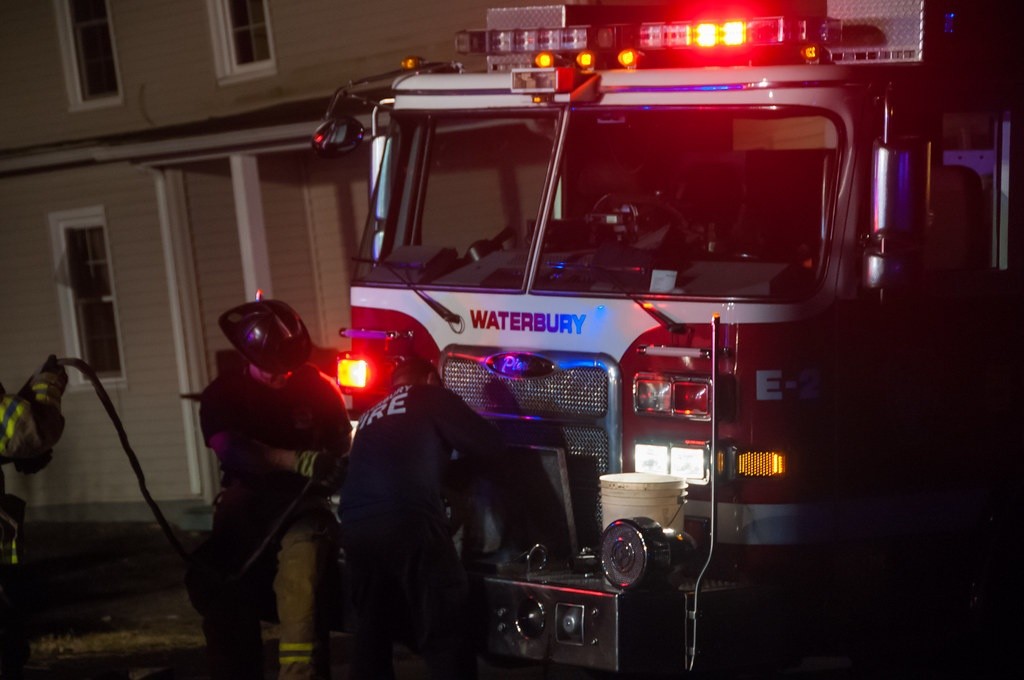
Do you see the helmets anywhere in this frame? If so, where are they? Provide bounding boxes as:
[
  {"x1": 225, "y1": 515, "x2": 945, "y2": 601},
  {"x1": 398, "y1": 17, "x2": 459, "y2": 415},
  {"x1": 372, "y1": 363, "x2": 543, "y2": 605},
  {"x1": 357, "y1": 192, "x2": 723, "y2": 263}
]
[{"x1": 218, "y1": 299, "x2": 312, "y2": 373}]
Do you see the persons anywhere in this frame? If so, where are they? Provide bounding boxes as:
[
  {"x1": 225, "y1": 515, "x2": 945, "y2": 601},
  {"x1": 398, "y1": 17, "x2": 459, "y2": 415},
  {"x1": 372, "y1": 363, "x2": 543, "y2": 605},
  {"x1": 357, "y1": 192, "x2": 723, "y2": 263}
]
[
  {"x1": 337, "y1": 362, "x2": 555, "y2": 680},
  {"x1": 184, "y1": 300, "x2": 351, "y2": 679},
  {"x1": 0, "y1": 355, "x2": 68, "y2": 680},
  {"x1": 925, "y1": 166, "x2": 991, "y2": 267}
]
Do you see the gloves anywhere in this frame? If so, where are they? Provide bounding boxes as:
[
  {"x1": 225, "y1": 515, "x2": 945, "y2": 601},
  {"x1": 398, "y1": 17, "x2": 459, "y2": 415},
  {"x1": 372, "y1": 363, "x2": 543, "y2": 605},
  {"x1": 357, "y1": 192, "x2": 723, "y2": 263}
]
[
  {"x1": 39, "y1": 354, "x2": 68, "y2": 386},
  {"x1": 290, "y1": 449, "x2": 337, "y2": 482}
]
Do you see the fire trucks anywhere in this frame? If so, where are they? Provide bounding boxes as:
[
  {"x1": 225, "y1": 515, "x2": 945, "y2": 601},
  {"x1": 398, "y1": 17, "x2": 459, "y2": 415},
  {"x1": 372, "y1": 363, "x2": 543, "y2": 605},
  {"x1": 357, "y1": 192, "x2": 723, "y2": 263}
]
[{"x1": 306, "y1": 1, "x2": 1024, "y2": 679}]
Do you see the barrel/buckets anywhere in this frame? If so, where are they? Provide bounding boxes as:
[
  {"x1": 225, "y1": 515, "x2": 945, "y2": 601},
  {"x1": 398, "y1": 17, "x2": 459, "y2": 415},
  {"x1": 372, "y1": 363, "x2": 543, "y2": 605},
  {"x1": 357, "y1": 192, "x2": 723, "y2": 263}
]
[{"x1": 600, "y1": 472, "x2": 689, "y2": 530}]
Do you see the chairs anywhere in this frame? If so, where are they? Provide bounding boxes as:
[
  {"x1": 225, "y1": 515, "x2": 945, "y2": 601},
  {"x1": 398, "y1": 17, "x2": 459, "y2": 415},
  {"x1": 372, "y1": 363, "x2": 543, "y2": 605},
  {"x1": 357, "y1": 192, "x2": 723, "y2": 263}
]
[{"x1": 928, "y1": 164, "x2": 986, "y2": 270}]
